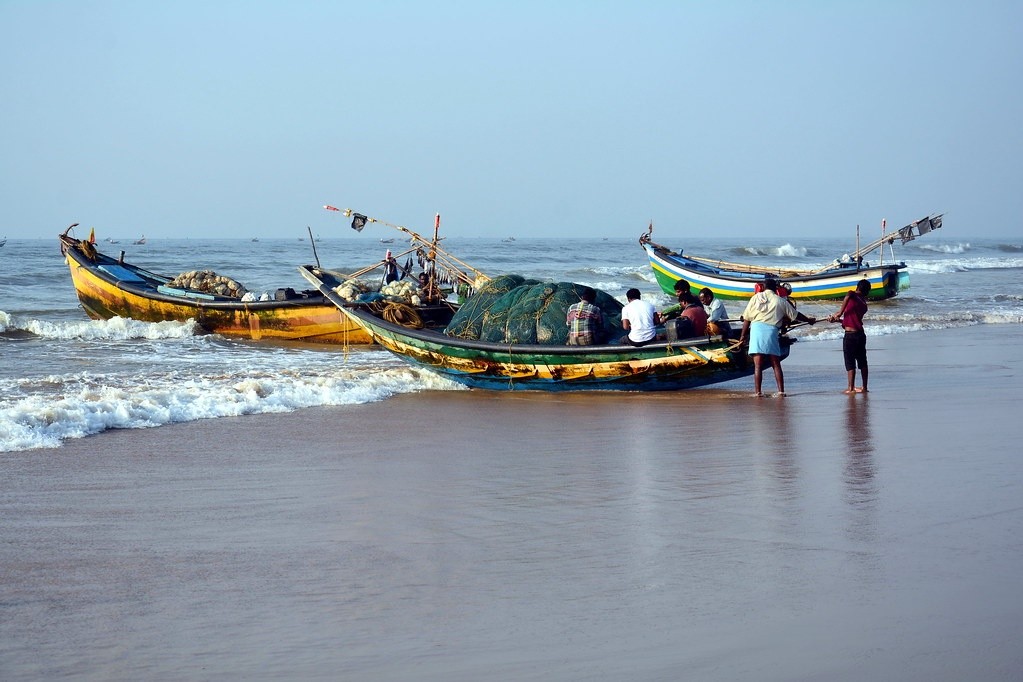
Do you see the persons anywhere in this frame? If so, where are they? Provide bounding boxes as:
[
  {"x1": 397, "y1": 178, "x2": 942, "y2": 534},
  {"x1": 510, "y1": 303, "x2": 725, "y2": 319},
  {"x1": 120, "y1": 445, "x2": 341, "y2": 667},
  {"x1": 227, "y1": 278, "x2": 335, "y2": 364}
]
[
  {"x1": 829, "y1": 279, "x2": 871, "y2": 394},
  {"x1": 567, "y1": 280, "x2": 732, "y2": 347},
  {"x1": 419, "y1": 252, "x2": 436, "y2": 280},
  {"x1": 417, "y1": 272, "x2": 440, "y2": 304},
  {"x1": 740, "y1": 278, "x2": 816, "y2": 397},
  {"x1": 456, "y1": 272, "x2": 474, "y2": 306}
]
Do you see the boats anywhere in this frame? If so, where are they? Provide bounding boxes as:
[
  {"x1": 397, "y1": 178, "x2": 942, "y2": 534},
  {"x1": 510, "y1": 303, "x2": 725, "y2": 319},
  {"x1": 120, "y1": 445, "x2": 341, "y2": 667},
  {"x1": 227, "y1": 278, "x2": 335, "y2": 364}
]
[
  {"x1": 57, "y1": 221, "x2": 378, "y2": 346},
  {"x1": 638, "y1": 211, "x2": 949, "y2": 302},
  {"x1": 110, "y1": 240, "x2": 121, "y2": 244},
  {"x1": 132, "y1": 234, "x2": 146, "y2": 245},
  {"x1": 297, "y1": 225, "x2": 798, "y2": 395},
  {"x1": 382, "y1": 237, "x2": 394, "y2": 244}
]
